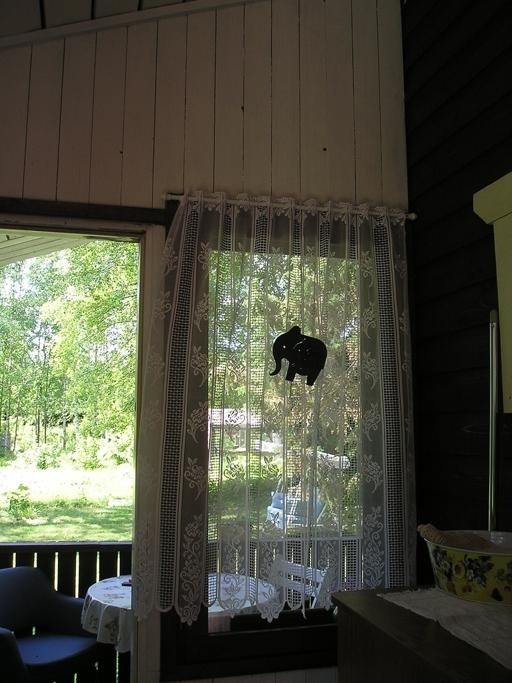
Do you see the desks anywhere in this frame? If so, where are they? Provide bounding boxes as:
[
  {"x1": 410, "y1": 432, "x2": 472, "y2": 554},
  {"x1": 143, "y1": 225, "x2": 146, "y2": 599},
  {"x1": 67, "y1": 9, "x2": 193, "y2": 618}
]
[
  {"x1": 330, "y1": 585, "x2": 512, "y2": 683},
  {"x1": 81, "y1": 571, "x2": 277, "y2": 683}
]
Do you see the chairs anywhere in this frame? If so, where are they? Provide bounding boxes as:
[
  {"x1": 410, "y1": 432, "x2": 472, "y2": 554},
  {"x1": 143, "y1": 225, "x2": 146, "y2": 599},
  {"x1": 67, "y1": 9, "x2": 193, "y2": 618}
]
[
  {"x1": 265, "y1": 554, "x2": 336, "y2": 609},
  {"x1": 0, "y1": 566, "x2": 108, "y2": 683}
]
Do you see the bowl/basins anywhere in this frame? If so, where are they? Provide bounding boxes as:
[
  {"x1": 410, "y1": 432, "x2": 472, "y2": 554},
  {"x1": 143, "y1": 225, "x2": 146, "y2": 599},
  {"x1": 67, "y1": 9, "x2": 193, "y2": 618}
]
[{"x1": 423, "y1": 529, "x2": 512, "y2": 604}]
